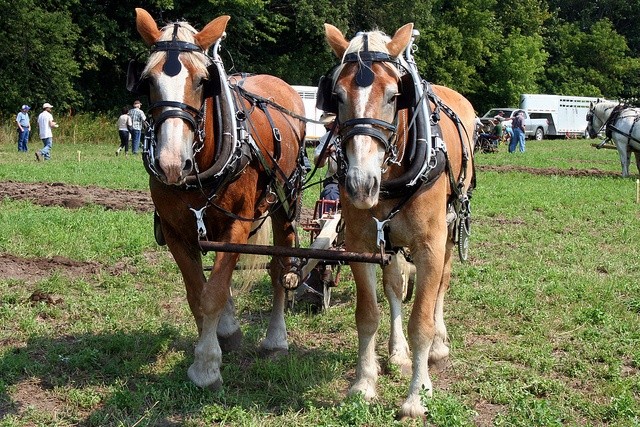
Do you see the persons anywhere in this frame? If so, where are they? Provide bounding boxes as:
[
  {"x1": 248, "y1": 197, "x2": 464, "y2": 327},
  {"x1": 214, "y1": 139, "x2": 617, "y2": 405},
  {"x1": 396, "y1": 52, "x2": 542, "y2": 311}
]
[
  {"x1": 494, "y1": 110, "x2": 514, "y2": 138},
  {"x1": 35, "y1": 103, "x2": 59, "y2": 160},
  {"x1": 115, "y1": 107, "x2": 132, "y2": 157},
  {"x1": 128, "y1": 100, "x2": 146, "y2": 154},
  {"x1": 314, "y1": 112, "x2": 339, "y2": 209},
  {"x1": 473, "y1": 112, "x2": 484, "y2": 147},
  {"x1": 16, "y1": 105, "x2": 32, "y2": 152},
  {"x1": 508, "y1": 110, "x2": 526, "y2": 153},
  {"x1": 480, "y1": 115, "x2": 503, "y2": 139},
  {"x1": 503, "y1": 124, "x2": 514, "y2": 142}
]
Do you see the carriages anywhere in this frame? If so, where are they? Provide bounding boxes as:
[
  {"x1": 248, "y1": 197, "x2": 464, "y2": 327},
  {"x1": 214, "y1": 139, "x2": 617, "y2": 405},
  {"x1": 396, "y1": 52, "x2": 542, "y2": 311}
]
[{"x1": 133, "y1": 7, "x2": 478, "y2": 417}]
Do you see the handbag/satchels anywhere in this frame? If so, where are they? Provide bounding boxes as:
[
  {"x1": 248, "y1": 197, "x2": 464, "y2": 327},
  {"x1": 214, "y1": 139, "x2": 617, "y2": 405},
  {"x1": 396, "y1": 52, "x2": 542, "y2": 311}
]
[{"x1": 127, "y1": 116, "x2": 133, "y2": 131}]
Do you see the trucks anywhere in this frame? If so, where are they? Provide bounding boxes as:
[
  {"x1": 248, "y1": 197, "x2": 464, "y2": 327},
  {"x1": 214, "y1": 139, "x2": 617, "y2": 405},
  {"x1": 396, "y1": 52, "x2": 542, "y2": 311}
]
[{"x1": 521, "y1": 94, "x2": 640, "y2": 139}]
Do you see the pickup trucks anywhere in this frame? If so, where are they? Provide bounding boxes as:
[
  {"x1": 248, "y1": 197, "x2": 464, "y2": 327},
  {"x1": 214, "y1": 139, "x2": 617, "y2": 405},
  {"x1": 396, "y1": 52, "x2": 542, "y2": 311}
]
[{"x1": 481, "y1": 108, "x2": 550, "y2": 139}]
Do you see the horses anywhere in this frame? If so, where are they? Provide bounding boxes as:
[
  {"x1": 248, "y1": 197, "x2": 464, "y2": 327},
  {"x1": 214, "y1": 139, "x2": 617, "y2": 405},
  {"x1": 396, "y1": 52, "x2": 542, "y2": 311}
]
[
  {"x1": 136, "y1": 7, "x2": 305, "y2": 392},
  {"x1": 324, "y1": 23, "x2": 476, "y2": 422},
  {"x1": 586, "y1": 98, "x2": 640, "y2": 178}
]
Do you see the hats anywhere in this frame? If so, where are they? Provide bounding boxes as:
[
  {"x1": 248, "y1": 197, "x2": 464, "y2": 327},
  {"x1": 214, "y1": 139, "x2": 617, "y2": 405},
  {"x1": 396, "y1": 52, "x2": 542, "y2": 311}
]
[
  {"x1": 42, "y1": 103, "x2": 54, "y2": 109},
  {"x1": 518, "y1": 110, "x2": 525, "y2": 113},
  {"x1": 134, "y1": 100, "x2": 142, "y2": 105},
  {"x1": 498, "y1": 111, "x2": 505, "y2": 114},
  {"x1": 22, "y1": 105, "x2": 31, "y2": 110}
]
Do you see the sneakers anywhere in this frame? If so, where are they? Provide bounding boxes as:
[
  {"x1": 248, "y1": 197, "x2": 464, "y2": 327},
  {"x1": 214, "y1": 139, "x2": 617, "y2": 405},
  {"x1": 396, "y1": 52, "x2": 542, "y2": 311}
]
[{"x1": 35, "y1": 150, "x2": 43, "y2": 162}]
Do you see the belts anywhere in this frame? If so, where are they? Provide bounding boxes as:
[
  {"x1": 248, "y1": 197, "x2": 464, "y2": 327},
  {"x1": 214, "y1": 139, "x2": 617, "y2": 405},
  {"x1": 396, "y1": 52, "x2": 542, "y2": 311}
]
[{"x1": 20, "y1": 125, "x2": 29, "y2": 128}]
